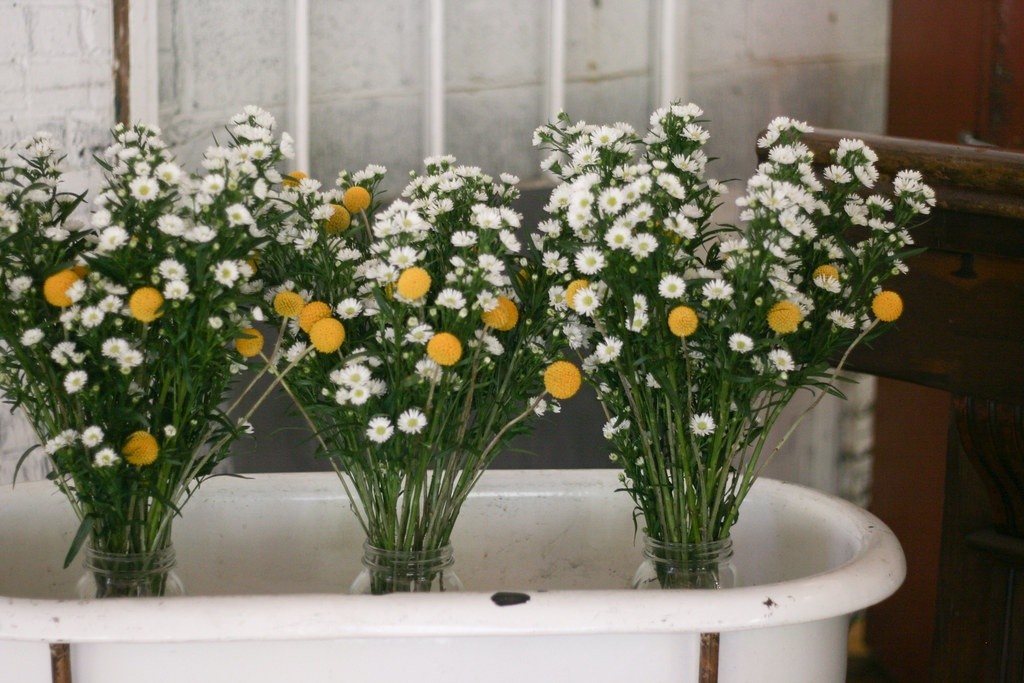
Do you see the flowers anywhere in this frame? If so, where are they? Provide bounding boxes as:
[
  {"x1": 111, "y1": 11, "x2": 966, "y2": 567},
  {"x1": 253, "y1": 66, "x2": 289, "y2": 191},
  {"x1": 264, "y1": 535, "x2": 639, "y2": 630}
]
[
  {"x1": 267, "y1": 167, "x2": 580, "y2": 547},
  {"x1": 530, "y1": 105, "x2": 934, "y2": 537},
  {"x1": 0, "y1": 105, "x2": 290, "y2": 550}
]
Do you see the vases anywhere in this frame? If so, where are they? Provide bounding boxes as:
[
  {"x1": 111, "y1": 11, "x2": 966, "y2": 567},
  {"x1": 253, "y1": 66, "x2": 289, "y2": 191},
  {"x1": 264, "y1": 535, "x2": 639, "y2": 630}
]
[
  {"x1": 72, "y1": 553, "x2": 187, "y2": 601},
  {"x1": 352, "y1": 542, "x2": 468, "y2": 595},
  {"x1": 634, "y1": 533, "x2": 739, "y2": 591}
]
